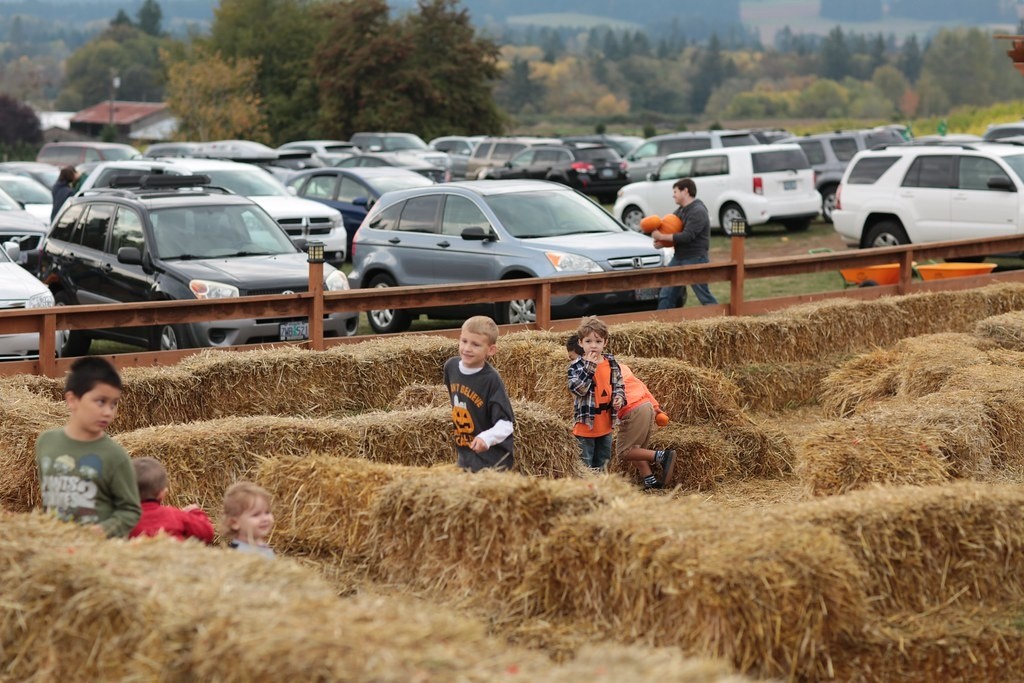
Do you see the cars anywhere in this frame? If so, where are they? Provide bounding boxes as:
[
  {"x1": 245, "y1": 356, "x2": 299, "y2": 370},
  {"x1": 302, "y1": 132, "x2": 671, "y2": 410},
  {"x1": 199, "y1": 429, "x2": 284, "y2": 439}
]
[
  {"x1": 0, "y1": 236, "x2": 63, "y2": 364},
  {"x1": 0, "y1": 174, "x2": 59, "y2": 227},
  {"x1": 338, "y1": 153, "x2": 445, "y2": 189},
  {"x1": 615, "y1": 143, "x2": 823, "y2": 239},
  {"x1": 0, "y1": 188, "x2": 45, "y2": 275},
  {"x1": 292, "y1": 165, "x2": 443, "y2": 265},
  {"x1": 0, "y1": 162, "x2": 60, "y2": 193},
  {"x1": 281, "y1": 141, "x2": 361, "y2": 168},
  {"x1": 427, "y1": 133, "x2": 489, "y2": 179}
]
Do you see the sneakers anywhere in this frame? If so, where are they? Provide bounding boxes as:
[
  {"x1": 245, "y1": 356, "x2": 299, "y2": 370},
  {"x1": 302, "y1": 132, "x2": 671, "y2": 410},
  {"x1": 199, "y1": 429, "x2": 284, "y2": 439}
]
[
  {"x1": 637, "y1": 483, "x2": 661, "y2": 491},
  {"x1": 661, "y1": 450, "x2": 677, "y2": 485}
]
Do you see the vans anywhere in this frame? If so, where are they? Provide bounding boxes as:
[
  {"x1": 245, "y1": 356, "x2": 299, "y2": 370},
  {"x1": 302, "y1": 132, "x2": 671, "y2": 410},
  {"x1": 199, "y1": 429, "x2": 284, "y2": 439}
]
[{"x1": 38, "y1": 142, "x2": 144, "y2": 171}]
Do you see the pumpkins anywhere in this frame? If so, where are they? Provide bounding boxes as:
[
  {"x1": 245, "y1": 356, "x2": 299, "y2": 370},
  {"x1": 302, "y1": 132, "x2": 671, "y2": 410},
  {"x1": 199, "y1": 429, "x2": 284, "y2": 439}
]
[{"x1": 641, "y1": 215, "x2": 683, "y2": 249}]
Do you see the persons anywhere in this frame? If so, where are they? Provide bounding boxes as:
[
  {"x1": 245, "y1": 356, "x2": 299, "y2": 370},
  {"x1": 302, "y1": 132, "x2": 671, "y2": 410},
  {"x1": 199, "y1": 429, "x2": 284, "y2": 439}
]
[
  {"x1": 68, "y1": 165, "x2": 88, "y2": 196},
  {"x1": 128, "y1": 457, "x2": 215, "y2": 545},
  {"x1": 442, "y1": 315, "x2": 516, "y2": 473},
  {"x1": 218, "y1": 479, "x2": 276, "y2": 559},
  {"x1": 568, "y1": 318, "x2": 627, "y2": 470},
  {"x1": 49, "y1": 167, "x2": 74, "y2": 222},
  {"x1": 656, "y1": 178, "x2": 718, "y2": 310},
  {"x1": 34, "y1": 356, "x2": 142, "y2": 540},
  {"x1": 567, "y1": 334, "x2": 678, "y2": 493}
]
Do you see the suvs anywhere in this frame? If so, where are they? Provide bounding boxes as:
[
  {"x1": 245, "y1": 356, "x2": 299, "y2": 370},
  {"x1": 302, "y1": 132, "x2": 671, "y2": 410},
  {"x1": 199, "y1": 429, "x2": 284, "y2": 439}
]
[
  {"x1": 350, "y1": 130, "x2": 448, "y2": 175},
  {"x1": 621, "y1": 129, "x2": 759, "y2": 197},
  {"x1": 41, "y1": 174, "x2": 359, "y2": 355},
  {"x1": 777, "y1": 128, "x2": 906, "y2": 224},
  {"x1": 153, "y1": 141, "x2": 330, "y2": 201},
  {"x1": 351, "y1": 178, "x2": 668, "y2": 338},
  {"x1": 465, "y1": 136, "x2": 563, "y2": 181},
  {"x1": 480, "y1": 141, "x2": 630, "y2": 205},
  {"x1": 834, "y1": 139, "x2": 1023, "y2": 269},
  {"x1": 73, "y1": 157, "x2": 348, "y2": 269}
]
